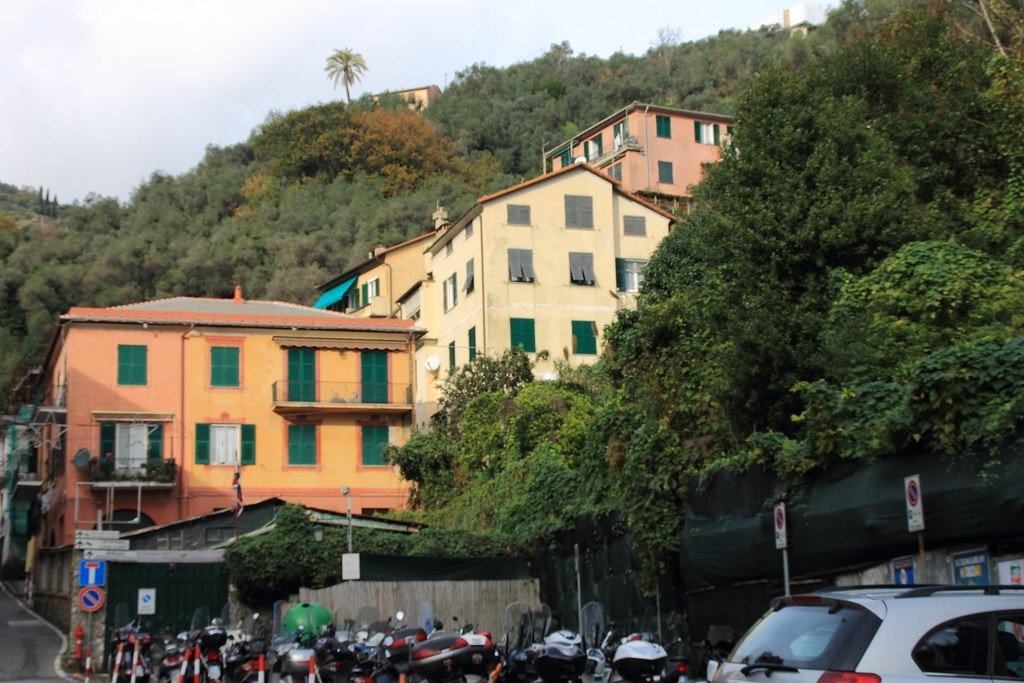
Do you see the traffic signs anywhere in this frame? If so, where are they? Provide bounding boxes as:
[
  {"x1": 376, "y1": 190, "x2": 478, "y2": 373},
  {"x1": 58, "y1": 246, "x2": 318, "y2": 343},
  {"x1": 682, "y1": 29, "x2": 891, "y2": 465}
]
[{"x1": 74, "y1": 531, "x2": 137, "y2": 562}]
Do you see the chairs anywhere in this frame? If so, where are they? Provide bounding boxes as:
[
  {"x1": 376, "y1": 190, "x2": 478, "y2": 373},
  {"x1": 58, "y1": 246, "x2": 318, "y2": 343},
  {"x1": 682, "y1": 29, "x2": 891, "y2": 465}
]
[{"x1": 998, "y1": 630, "x2": 1022, "y2": 677}]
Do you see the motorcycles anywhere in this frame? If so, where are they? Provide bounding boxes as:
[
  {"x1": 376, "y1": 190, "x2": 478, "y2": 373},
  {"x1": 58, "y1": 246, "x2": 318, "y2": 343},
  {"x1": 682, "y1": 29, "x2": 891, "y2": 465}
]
[{"x1": 108, "y1": 603, "x2": 707, "y2": 683}]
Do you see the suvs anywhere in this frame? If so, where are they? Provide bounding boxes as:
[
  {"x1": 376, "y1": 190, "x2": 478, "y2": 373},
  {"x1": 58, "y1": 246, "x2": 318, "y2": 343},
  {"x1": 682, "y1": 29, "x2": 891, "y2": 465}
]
[{"x1": 711, "y1": 585, "x2": 1024, "y2": 683}]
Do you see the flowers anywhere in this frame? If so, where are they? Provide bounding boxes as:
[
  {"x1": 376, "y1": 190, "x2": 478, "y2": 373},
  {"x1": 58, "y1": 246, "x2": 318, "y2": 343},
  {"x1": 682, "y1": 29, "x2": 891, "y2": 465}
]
[{"x1": 99, "y1": 452, "x2": 114, "y2": 472}]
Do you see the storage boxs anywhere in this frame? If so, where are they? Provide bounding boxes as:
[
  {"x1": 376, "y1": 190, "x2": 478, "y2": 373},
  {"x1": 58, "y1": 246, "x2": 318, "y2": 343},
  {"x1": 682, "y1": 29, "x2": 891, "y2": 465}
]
[
  {"x1": 198, "y1": 625, "x2": 227, "y2": 649},
  {"x1": 386, "y1": 627, "x2": 428, "y2": 658},
  {"x1": 408, "y1": 636, "x2": 472, "y2": 676},
  {"x1": 285, "y1": 647, "x2": 316, "y2": 673},
  {"x1": 462, "y1": 633, "x2": 494, "y2": 665},
  {"x1": 612, "y1": 640, "x2": 669, "y2": 678},
  {"x1": 534, "y1": 643, "x2": 587, "y2": 681}
]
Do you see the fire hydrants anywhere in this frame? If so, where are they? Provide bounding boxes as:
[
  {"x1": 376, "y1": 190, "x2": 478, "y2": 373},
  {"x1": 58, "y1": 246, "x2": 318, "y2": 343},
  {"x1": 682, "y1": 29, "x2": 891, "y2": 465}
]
[{"x1": 74, "y1": 624, "x2": 84, "y2": 673}]
